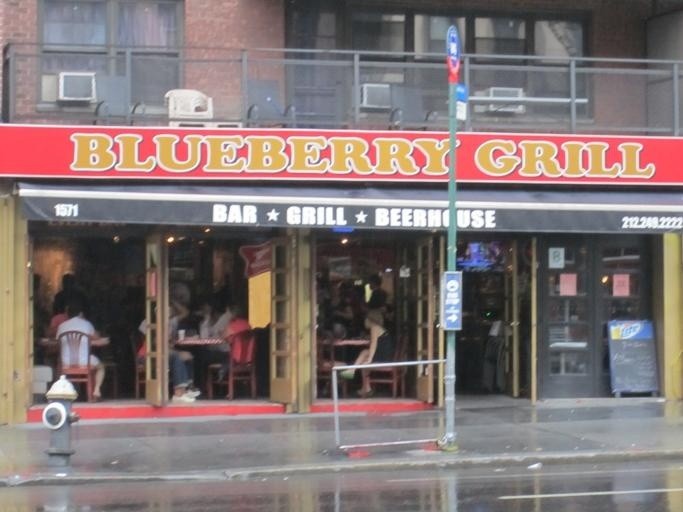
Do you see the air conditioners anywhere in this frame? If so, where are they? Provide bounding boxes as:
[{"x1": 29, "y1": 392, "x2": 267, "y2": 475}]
[
  {"x1": 54, "y1": 69, "x2": 94, "y2": 104},
  {"x1": 482, "y1": 86, "x2": 523, "y2": 112},
  {"x1": 357, "y1": 83, "x2": 389, "y2": 112}
]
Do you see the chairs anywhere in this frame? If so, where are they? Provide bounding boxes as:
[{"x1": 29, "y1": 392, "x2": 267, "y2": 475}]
[
  {"x1": 90, "y1": 73, "x2": 147, "y2": 125},
  {"x1": 386, "y1": 85, "x2": 441, "y2": 131},
  {"x1": 164, "y1": 86, "x2": 216, "y2": 127},
  {"x1": 239, "y1": 76, "x2": 298, "y2": 128}
]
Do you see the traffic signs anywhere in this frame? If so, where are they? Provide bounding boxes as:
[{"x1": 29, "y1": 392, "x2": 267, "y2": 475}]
[{"x1": 443, "y1": 271, "x2": 463, "y2": 331}]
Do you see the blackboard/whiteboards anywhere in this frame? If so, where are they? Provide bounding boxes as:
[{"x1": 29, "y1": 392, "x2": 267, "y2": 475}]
[{"x1": 607, "y1": 319, "x2": 659, "y2": 394}]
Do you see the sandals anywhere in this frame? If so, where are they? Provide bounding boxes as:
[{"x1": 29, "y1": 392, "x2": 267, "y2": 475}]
[{"x1": 339, "y1": 370, "x2": 355, "y2": 379}]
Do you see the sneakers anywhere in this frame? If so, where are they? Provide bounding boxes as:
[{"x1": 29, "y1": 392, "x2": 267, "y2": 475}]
[{"x1": 171, "y1": 389, "x2": 201, "y2": 404}]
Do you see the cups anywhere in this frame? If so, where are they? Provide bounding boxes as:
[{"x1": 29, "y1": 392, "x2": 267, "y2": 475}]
[{"x1": 178, "y1": 329, "x2": 185, "y2": 341}]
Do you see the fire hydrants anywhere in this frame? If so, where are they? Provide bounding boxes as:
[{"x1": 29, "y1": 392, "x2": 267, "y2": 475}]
[{"x1": 39, "y1": 372, "x2": 81, "y2": 470}]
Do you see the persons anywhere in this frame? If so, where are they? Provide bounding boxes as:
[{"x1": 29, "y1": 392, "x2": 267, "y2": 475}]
[{"x1": 42, "y1": 274, "x2": 398, "y2": 404}]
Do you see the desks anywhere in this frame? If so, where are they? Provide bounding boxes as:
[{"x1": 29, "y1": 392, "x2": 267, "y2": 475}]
[{"x1": 454, "y1": 94, "x2": 588, "y2": 136}]
